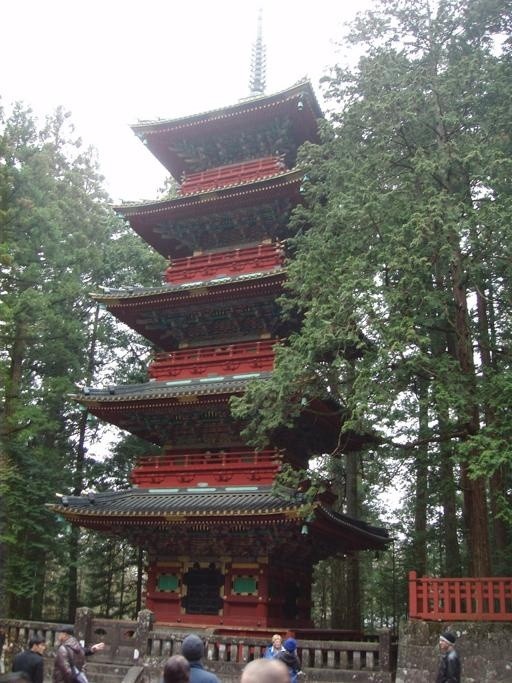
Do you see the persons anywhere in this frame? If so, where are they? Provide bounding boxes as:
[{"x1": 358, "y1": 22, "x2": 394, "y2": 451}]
[
  {"x1": 82, "y1": 642, "x2": 105, "y2": 656},
  {"x1": 241, "y1": 657, "x2": 292, "y2": 683},
  {"x1": 12, "y1": 636, "x2": 47, "y2": 683},
  {"x1": 276, "y1": 637, "x2": 303, "y2": 683},
  {"x1": 52, "y1": 626, "x2": 88, "y2": 683},
  {"x1": 180, "y1": 634, "x2": 219, "y2": 683},
  {"x1": 161, "y1": 654, "x2": 192, "y2": 683},
  {"x1": 435, "y1": 632, "x2": 461, "y2": 682},
  {"x1": 262, "y1": 634, "x2": 284, "y2": 659},
  {"x1": 0, "y1": 670, "x2": 31, "y2": 683}
]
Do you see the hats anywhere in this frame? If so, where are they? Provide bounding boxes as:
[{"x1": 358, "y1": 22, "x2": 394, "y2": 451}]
[
  {"x1": 57, "y1": 625, "x2": 74, "y2": 632},
  {"x1": 271, "y1": 634, "x2": 282, "y2": 643},
  {"x1": 282, "y1": 638, "x2": 296, "y2": 652},
  {"x1": 181, "y1": 634, "x2": 204, "y2": 660},
  {"x1": 440, "y1": 632, "x2": 455, "y2": 645}
]
